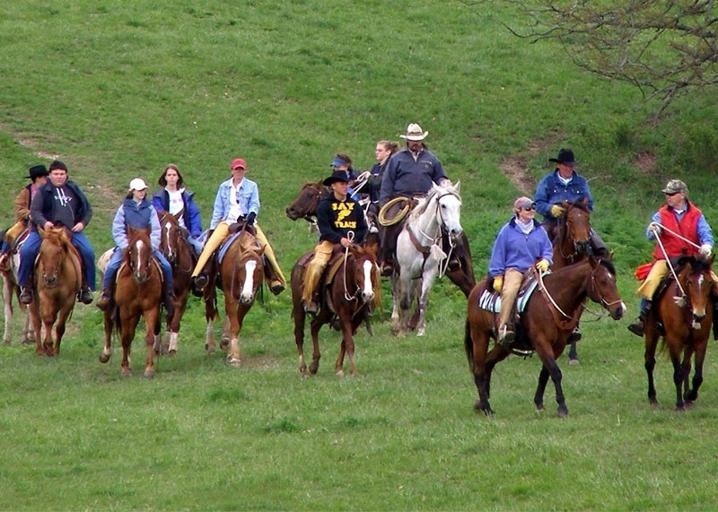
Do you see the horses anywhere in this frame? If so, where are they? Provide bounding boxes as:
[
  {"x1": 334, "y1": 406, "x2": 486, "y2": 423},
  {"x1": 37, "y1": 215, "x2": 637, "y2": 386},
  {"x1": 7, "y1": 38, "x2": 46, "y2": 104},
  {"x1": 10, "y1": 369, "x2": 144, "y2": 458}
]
[
  {"x1": 152, "y1": 207, "x2": 195, "y2": 358},
  {"x1": 644, "y1": 253, "x2": 716, "y2": 411},
  {"x1": 205, "y1": 230, "x2": 267, "y2": 367},
  {"x1": 99, "y1": 223, "x2": 162, "y2": 379},
  {"x1": 285, "y1": 176, "x2": 476, "y2": 379},
  {"x1": 548, "y1": 196, "x2": 593, "y2": 366},
  {"x1": 0, "y1": 217, "x2": 83, "y2": 357},
  {"x1": 464, "y1": 253, "x2": 626, "y2": 417}
]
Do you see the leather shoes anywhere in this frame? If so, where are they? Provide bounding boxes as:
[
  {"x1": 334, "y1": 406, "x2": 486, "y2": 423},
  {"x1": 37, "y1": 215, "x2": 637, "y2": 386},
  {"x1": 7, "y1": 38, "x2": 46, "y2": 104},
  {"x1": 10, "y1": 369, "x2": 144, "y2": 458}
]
[
  {"x1": 194, "y1": 275, "x2": 207, "y2": 297},
  {"x1": 497, "y1": 328, "x2": 518, "y2": 346},
  {"x1": 164, "y1": 287, "x2": 181, "y2": 307},
  {"x1": 628, "y1": 323, "x2": 644, "y2": 337},
  {"x1": 448, "y1": 255, "x2": 459, "y2": 270},
  {"x1": 271, "y1": 279, "x2": 285, "y2": 295},
  {"x1": 381, "y1": 261, "x2": 392, "y2": 276},
  {"x1": 77, "y1": 289, "x2": 92, "y2": 304},
  {"x1": 302, "y1": 300, "x2": 318, "y2": 313},
  {"x1": 21, "y1": 288, "x2": 32, "y2": 304},
  {"x1": 567, "y1": 328, "x2": 582, "y2": 343},
  {"x1": 96, "y1": 288, "x2": 112, "y2": 310}
]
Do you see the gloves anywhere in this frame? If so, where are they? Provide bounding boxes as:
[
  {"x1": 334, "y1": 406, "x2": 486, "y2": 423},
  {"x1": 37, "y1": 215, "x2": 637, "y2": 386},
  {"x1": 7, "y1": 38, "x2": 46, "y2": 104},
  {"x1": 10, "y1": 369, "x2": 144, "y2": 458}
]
[
  {"x1": 247, "y1": 212, "x2": 256, "y2": 225},
  {"x1": 551, "y1": 205, "x2": 564, "y2": 218},
  {"x1": 698, "y1": 243, "x2": 712, "y2": 257},
  {"x1": 493, "y1": 275, "x2": 503, "y2": 292},
  {"x1": 536, "y1": 259, "x2": 549, "y2": 271}
]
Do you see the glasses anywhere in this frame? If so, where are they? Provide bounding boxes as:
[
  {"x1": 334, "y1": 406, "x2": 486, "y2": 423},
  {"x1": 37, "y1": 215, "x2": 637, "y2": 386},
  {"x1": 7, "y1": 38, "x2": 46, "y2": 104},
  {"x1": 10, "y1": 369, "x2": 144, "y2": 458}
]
[{"x1": 526, "y1": 208, "x2": 535, "y2": 211}]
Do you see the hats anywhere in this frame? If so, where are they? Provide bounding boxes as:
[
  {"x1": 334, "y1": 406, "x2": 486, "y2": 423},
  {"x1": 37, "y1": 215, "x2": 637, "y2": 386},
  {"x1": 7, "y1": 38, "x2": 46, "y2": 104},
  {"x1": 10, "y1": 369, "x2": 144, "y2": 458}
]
[
  {"x1": 514, "y1": 196, "x2": 534, "y2": 208},
  {"x1": 661, "y1": 180, "x2": 689, "y2": 194},
  {"x1": 24, "y1": 166, "x2": 49, "y2": 179},
  {"x1": 549, "y1": 149, "x2": 580, "y2": 165},
  {"x1": 323, "y1": 170, "x2": 349, "y2": 186},
  {"x1": 400, "y1": 123, "x2": 429, "y2": 141},
  {"x1": 128, "y1": 178, "x2": 149, "y2": 192},
  {"x1": 231, "y1": 159, "x2": 246, "y2": 170},
  {"x1": 330, "y1": 158, "x2": 346, "y2": 168}
]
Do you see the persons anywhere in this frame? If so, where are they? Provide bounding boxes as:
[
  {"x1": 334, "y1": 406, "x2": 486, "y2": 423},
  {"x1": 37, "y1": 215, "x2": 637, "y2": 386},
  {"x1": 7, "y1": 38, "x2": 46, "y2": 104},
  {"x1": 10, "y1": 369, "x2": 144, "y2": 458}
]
[
  {"x1": 533, "y1": 148, "x2": 610, "y2": 249},
  {"x1": 628, "y1": 177, "x2": 717, "y2": 340},
  {"x1": 16, "y1": 159, "x2": 96, "y2": 304},
  {"x1": 300, "y1": 122, "x2": 461, "y2": 313},
  {"x1": 152, "y1": 163, "x2": 202, "y2": 255},
  {"x1": 488, "y1": 197, "x2": 581, "y2": 347},
  {"x1": 96, "y1": 177, "x2": 181, "y2": 308},
  {"x1": 0, "y1": 164, "x2": 49, "y2": 272},
  {"x1": 190, "y1": 158, "x2": 284, "y2": 297}
]
